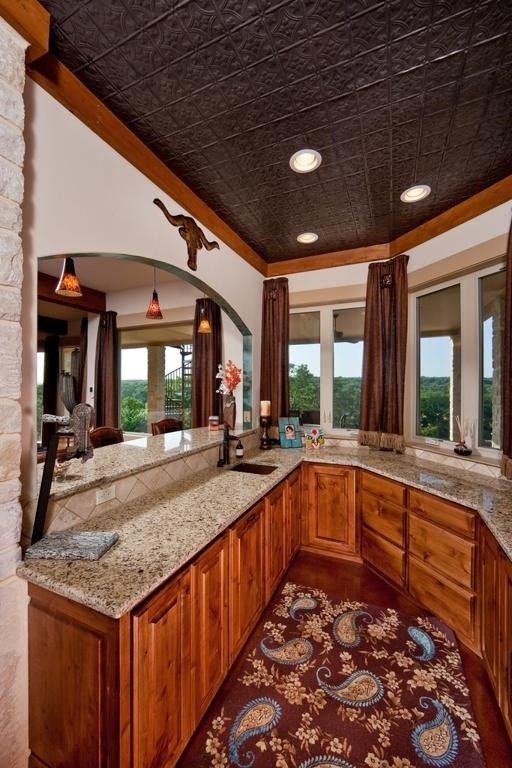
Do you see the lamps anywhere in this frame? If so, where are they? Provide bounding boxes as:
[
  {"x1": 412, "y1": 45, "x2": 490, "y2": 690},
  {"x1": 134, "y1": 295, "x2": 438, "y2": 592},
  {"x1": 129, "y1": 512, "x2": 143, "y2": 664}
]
[{"x1": 55, "y1": 255, "x2": 214, "y2": 334}]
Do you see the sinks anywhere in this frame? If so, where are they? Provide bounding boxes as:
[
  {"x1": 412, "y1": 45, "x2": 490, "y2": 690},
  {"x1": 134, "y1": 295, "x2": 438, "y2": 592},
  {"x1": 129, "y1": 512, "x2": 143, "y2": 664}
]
[{"x1": 227, "y1": 463, "x2": 279, "y2": 475}]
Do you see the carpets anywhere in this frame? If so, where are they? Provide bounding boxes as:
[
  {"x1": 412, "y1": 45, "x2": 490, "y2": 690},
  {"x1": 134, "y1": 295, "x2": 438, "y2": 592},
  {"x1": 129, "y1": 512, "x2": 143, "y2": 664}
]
[{"x1": 191, "y1": 576, "x2": 488, "y2": 767}]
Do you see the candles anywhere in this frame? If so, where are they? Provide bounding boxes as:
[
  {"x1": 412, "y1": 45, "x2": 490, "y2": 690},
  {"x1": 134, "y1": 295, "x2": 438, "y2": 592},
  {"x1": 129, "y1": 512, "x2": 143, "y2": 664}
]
[{"x1": 259, "y1": 400, "x2": 272, "y2": 417}]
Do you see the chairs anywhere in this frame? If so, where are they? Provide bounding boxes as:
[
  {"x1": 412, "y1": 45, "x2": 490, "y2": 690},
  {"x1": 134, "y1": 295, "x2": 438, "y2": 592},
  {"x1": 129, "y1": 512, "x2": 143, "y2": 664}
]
[{"x1": 89, "y1": 418, "x2": 184, "y2": 450}]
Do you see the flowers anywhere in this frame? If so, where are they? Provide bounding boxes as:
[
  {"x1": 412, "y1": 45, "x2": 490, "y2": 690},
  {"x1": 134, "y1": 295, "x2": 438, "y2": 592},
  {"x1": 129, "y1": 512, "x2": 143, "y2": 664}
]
[{"x1": 214, "y1": 359, "x2": 241, "y2": 396}]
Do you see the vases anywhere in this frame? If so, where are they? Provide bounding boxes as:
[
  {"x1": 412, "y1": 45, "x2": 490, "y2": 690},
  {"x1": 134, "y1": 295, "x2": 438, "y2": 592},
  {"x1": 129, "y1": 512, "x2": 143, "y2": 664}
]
[{"x1": 222, "y1": 393, "x2": 237, "y2": 431}]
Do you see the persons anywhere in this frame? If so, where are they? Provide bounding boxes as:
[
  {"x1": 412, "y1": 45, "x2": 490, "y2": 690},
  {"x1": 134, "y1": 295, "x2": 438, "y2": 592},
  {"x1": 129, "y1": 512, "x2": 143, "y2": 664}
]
[{"x1": 284, "y1": 426, "x2": 296, "y2": 439}]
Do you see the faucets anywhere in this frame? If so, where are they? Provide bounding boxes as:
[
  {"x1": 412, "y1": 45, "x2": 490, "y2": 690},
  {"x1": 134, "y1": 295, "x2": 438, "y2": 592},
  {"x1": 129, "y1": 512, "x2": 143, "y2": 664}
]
[{"x1": 224, "y1": 422, "x2": 238, "y2": 465}]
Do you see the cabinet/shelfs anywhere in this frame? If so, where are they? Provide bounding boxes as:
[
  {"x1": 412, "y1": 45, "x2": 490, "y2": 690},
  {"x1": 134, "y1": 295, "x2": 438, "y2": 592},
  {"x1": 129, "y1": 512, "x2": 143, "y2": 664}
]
[
  {"x1": 228, "y1": 499, "x2": 266, "y2": 671},
  {"x1": 30, "y1": 535, "x2": 229, "y2": 768},
  {"x1": 266, "y1": 467, "x2": 303, "y2": 613},
  {"x1": 482, "y1": 525, "x2": 512, "y2": 745},
  {"x1": 307, "y1": 464, "x2": 357, "y2": 563},
  {"x1": 359, "y1": 469, "x2": 481, "y2": 659}
]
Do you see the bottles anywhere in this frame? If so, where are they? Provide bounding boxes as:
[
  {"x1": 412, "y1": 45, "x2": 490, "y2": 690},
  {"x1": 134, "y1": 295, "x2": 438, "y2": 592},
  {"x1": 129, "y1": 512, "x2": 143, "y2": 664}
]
[
  {"x1": 236, "y1": 440, "x2": 244, "y2": 458},
  {"x1": 209, "y1": 415, "x2": 219, "y2": 431}
]
